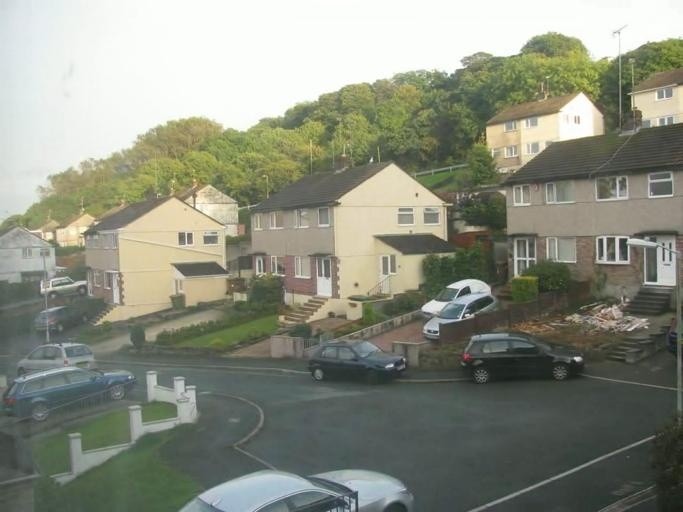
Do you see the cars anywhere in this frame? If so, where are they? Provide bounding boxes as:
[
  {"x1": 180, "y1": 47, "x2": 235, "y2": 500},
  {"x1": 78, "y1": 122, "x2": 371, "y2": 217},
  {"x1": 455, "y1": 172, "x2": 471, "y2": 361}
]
[
  {"x1": 422, "y1": 292, "x2": 500, "y2": 340},
  {"x1": 665, "y1": 315, "x2": 682, "y2": 361},
  {"x1": 176, "y1": 468, "x2": 415, "y2": 512},
  {"x1": 420, "y1": 279, "x2": 492, "y2": 320}
]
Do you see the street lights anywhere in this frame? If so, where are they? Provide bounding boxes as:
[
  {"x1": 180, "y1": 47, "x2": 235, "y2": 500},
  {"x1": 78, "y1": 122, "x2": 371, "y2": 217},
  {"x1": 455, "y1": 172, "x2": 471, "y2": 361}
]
[
  {"x1": 628, "y1": 58, "x2": 638, "y2": 133},
  {"x1": 624, "y1": 237, "x2": 682, "y2": 256},
  {"x1": 261, "y1": 174, "x2": 268, "y2": 199}
]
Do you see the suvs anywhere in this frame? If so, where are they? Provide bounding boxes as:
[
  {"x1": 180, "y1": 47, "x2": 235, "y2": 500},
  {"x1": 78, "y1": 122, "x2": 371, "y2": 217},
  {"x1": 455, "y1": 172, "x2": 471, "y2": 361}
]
[
  {"x1": 308, "y1": 337, "x2": 408, "y2": 385},
  {"x1": 462, "y1": 329, "x2": 584, "y2": 385},
  {"x1": 41, "y1": 277, "x2": 88, "y2": 300},
  {"x1": 4, "y1": 367, "x2": 137, "y2": 422},
  {"x1": 17, "y1": 343, "x2": 96, "y2": 376},
  {"x1": 34, "y1": 306, "x2": 89, "y2": 335}
]
